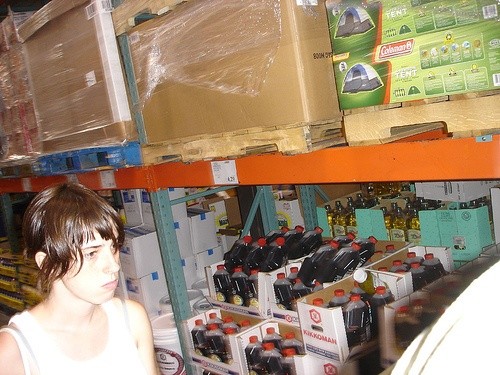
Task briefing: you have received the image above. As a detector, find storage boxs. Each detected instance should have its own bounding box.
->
[0,0,500,375]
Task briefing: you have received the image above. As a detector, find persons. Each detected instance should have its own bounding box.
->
[0,182,163,375]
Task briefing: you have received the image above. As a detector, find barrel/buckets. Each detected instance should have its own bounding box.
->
[151,312,187,375]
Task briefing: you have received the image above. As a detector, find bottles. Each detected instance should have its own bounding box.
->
[325,184,494,247]
[192,223,447,375]
